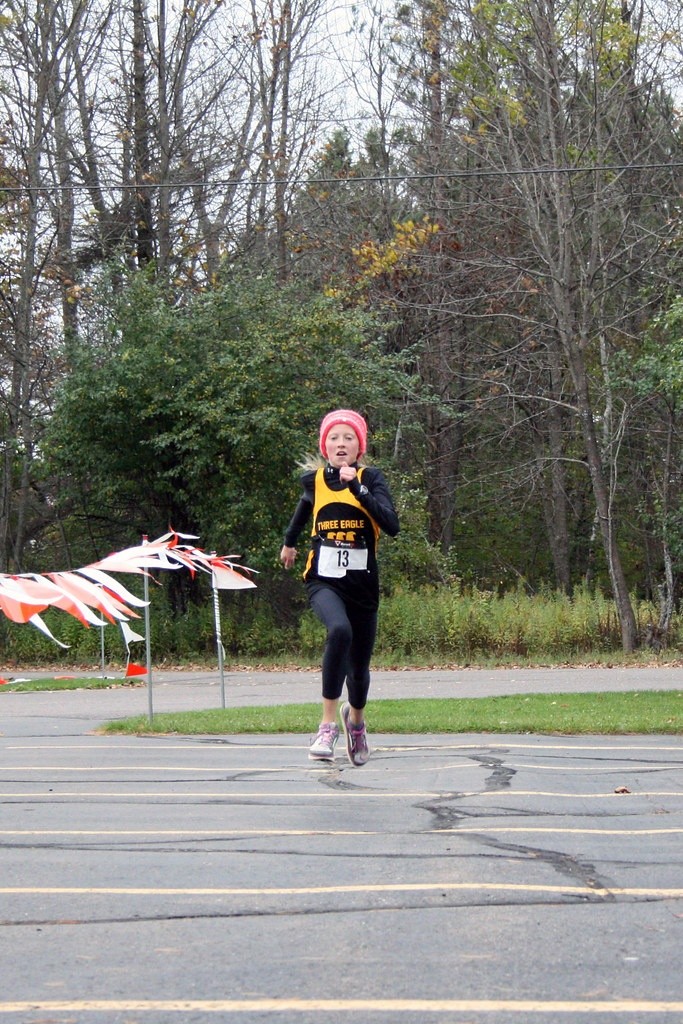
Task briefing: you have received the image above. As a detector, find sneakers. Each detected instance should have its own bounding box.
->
[307,721,339,762]
[340,700,371,768]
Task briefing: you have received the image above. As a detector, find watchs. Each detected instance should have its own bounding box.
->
[356,485,369,500]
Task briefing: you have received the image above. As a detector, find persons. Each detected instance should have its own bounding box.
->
[280,409,400,766]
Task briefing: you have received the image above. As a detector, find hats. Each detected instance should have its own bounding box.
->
[319,409,368,461]
